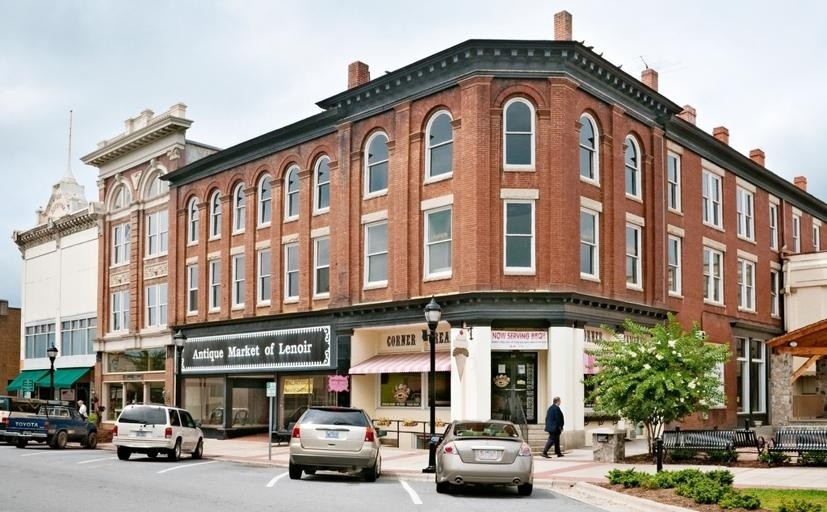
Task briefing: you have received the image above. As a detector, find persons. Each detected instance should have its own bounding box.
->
[77,400,88,422]
[541,396,565,459]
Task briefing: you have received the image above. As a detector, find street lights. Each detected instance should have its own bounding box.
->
[421,293,442,474]
[169,328,188,409]
[45,342,58,402]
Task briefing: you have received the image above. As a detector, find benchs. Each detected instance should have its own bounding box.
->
[652,430,736,466]
[736,430,766,456]
[767,430,827,468]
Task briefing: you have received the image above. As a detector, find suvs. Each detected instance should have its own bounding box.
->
[287,404,383,483]
[112,404,205,462]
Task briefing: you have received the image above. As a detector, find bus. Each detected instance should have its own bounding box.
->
[0,395,73,446]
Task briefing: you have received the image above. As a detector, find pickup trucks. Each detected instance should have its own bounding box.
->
[5,406,97,451]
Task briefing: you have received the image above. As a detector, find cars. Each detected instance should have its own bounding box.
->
[282,406,308,431]
[430,420,534,497]
